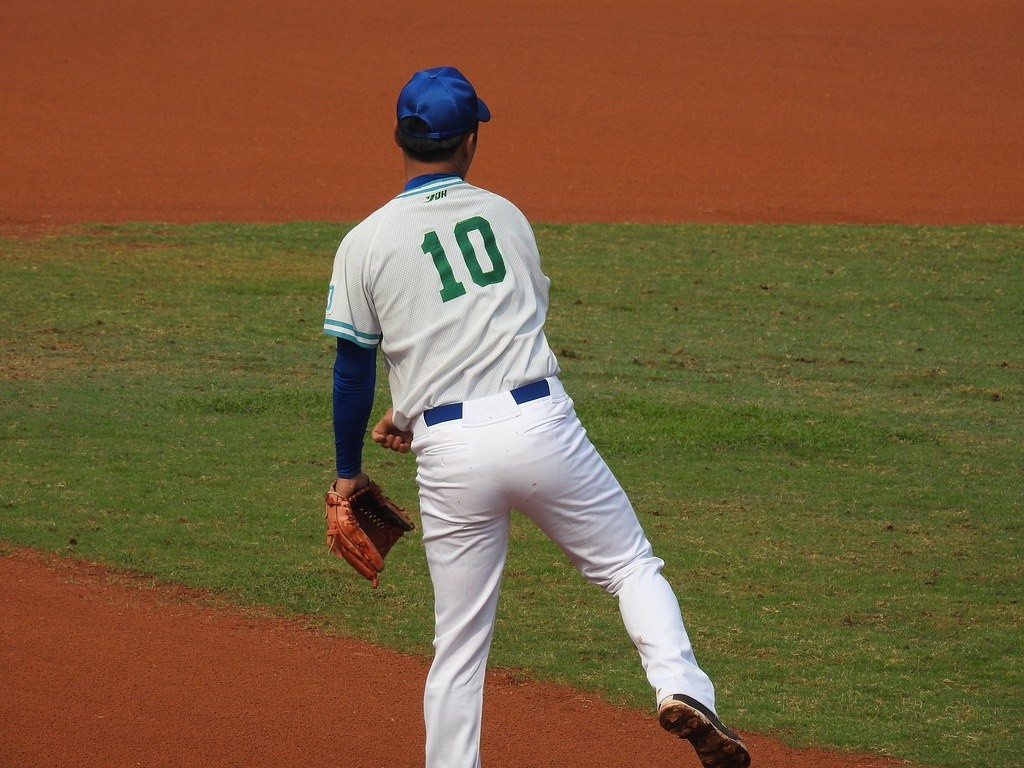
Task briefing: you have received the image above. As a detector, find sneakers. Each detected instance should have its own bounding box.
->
[657,694,751,768]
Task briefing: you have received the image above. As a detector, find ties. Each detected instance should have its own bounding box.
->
[397,65,491,138]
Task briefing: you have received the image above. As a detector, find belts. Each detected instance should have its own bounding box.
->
[424,377,549,427]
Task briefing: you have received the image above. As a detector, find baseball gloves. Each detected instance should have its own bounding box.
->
[322,476,417,589]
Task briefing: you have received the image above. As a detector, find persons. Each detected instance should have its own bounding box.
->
[324,67,751,768]
